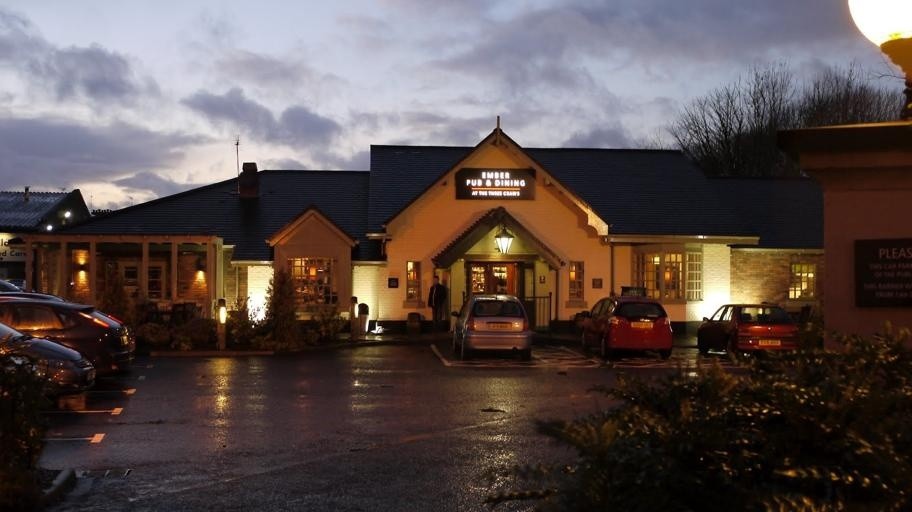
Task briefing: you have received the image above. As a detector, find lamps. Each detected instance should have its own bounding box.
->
[495,226,515,255]
[214,299,227,325]
[80,265,85,271]
[198,265,204,271]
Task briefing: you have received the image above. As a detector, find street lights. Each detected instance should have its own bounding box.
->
[837,1,912,130]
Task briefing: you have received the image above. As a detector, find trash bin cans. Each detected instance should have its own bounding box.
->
[407,312,420,335]
[359,303,369,334]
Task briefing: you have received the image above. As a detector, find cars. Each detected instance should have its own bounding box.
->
[1,279,138,493]
[452,291,531,359]
[575,294,676,361]
[695,301,798,356]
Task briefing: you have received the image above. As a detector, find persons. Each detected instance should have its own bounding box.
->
[428,275,449,332]
